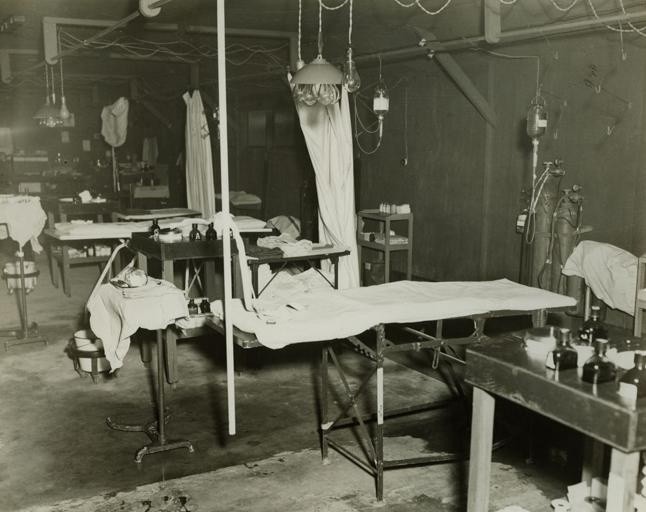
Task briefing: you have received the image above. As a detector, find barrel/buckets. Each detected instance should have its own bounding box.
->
[5,262,37,290]
[69,329,112,374]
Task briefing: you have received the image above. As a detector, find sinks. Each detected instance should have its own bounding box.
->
[610,340,646,374]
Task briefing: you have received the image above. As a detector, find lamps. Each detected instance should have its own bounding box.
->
[285,0,360,106]
[31,26,69,129]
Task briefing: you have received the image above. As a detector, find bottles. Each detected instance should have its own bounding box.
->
[582,337,616,384]
[189,223,202,242]
[618,350,645,411]
[206,224,217,240]
[150,219,160,235]
[372,87,390,120]
[545,328,578,371]
[187,298,199,315]
[577,304,609,346]
[526,104,547,145]
[200,299,211,313]
